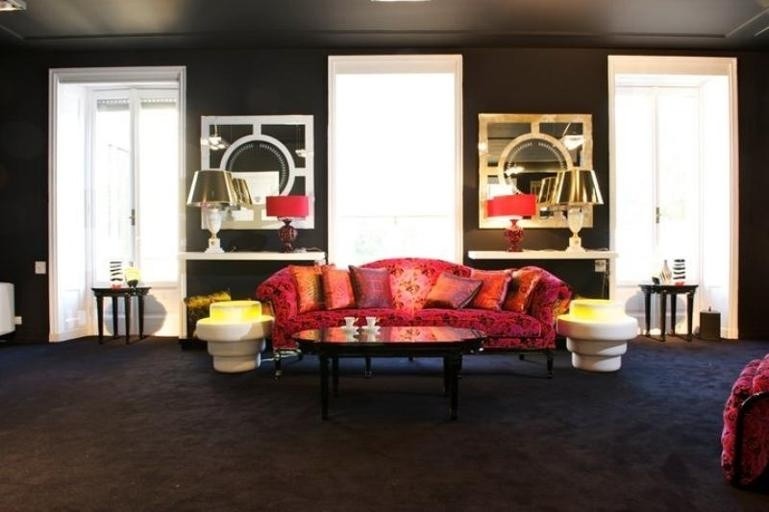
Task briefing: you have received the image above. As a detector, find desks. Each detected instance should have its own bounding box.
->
[90,285,151,345]
[637,281,700,342]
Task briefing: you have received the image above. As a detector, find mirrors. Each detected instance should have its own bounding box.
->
[200,112,316,233]
[476,111,597,232]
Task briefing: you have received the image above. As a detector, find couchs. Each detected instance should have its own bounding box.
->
[255,255,572,381]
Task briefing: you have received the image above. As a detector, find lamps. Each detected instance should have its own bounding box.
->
[185,167,240,253]
[264,194,310,252]
[484,193,539,251]
[226,178,254,224]
[196,298,275,377]
[555,298,640,375]
[538,175,566,222]
[544,167,604,253]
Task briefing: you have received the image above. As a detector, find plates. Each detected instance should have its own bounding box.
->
[361,324,381,332]
[341,325,358,332]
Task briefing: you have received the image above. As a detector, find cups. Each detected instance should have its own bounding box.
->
[366,316,380,327]
[343,315,358,327]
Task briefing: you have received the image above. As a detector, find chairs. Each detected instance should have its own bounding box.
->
[718,353,769,512]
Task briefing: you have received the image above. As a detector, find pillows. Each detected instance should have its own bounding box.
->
[288,262,324,317]
[470,268,514,313]
[424,271,484,310]
[348,264,398,310]
[319,264,358,311]
[502,268,544,314]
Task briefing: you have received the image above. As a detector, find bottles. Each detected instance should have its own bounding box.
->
[124,262,140,288]
